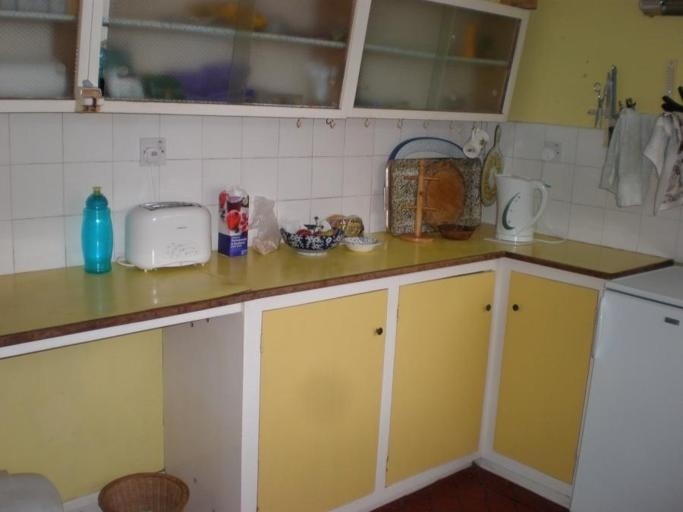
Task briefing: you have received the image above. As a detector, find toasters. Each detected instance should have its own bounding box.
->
[120,199,212,271]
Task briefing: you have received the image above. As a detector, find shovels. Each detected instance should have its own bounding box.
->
[481,126,505,205]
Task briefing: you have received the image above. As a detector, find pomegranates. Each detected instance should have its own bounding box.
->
[227,209,241,229]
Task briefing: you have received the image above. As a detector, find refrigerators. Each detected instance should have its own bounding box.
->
[567,265,683,512]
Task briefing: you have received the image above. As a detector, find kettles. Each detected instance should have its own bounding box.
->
[493,174,549,242]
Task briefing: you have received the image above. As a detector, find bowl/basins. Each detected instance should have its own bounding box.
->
[437,223,476,241]
[278,225,343,257]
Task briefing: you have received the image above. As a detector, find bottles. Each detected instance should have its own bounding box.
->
[78,184,115,274]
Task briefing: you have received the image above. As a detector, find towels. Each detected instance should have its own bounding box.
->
[600,109,683,214]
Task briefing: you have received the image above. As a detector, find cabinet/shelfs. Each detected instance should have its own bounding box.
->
[1,4,91,107]
[491,263,600,507]
[383,263,496,499]
[90,3,359,114]
[347,3,527,115]
[248,286,389,507]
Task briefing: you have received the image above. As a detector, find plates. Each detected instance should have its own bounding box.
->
[342,237,382,252]
[416,160,466,231]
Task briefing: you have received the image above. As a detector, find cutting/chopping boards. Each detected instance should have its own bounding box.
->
[384,156,484,236]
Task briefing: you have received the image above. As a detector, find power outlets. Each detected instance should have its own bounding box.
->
[543,141,561,164]
[139,137,167,166]
